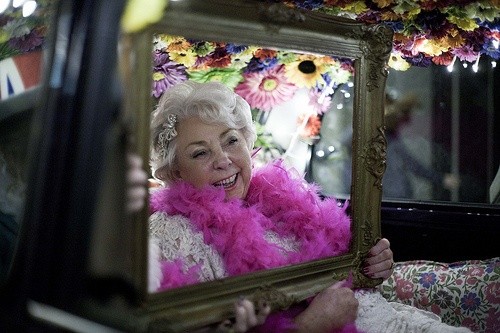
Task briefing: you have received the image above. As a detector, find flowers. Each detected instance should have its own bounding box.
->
[0,0,500,155]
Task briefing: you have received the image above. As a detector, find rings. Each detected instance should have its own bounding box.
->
[390,263,395,271]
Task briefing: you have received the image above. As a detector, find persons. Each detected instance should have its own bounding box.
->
[149,81,500,333]
[384,83,458,200]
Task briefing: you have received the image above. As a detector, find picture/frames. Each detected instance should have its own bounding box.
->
[116,0,395,333]
